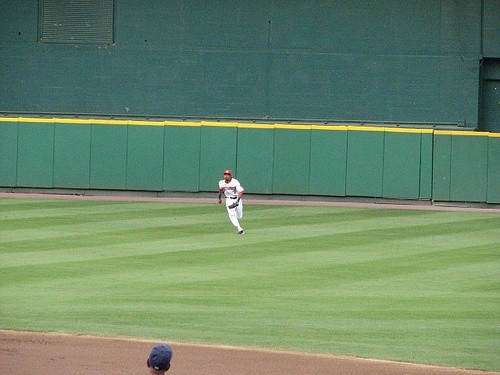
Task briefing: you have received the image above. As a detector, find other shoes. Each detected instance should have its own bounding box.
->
[239,228,243,233]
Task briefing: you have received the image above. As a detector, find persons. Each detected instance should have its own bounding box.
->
[218,170,246,235]
[147,344,173,375]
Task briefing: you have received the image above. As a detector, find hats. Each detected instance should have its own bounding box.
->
[149,344,172,368]
[224,170,232,174]
